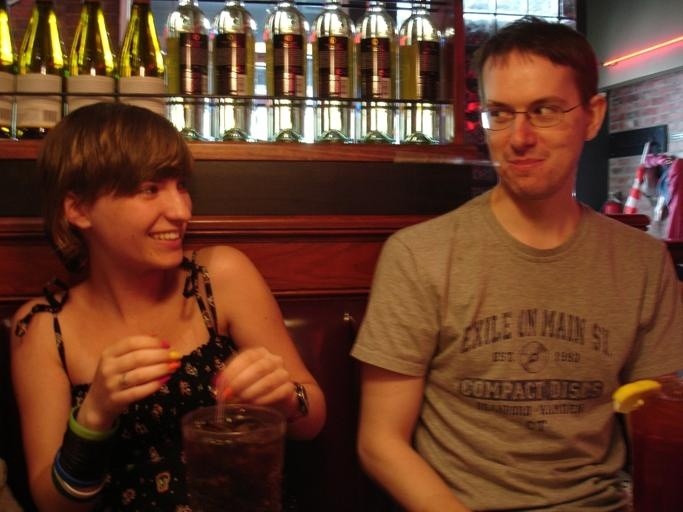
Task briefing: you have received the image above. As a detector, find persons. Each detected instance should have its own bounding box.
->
[357,16,683,507]
[8,102,328,506]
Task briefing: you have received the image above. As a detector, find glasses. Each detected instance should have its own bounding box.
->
[478,95,588,132]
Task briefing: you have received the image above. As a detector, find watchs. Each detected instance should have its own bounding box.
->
[293,381,312,416]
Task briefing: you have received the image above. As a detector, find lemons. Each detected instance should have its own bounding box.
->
[611,380,662,413]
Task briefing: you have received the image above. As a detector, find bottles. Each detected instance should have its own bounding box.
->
[312,0,356,145]
[0,0,19,139]
[360,0,396,146]
[70,1,115,117]
[264,0,307,144]
[118,0,165,121]
[399,0,442,145]
[210,0,257,144]
[162,0,210,143]
[16,0,65,141]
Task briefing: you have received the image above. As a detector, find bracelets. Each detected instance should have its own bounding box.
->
[67,407,117,444]
[53,454,112,500]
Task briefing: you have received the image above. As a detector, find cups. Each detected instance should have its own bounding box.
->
[631,376,683,512]
[181,404,287,512]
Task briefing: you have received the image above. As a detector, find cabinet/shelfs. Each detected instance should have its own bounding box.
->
[0,1,479,167]
